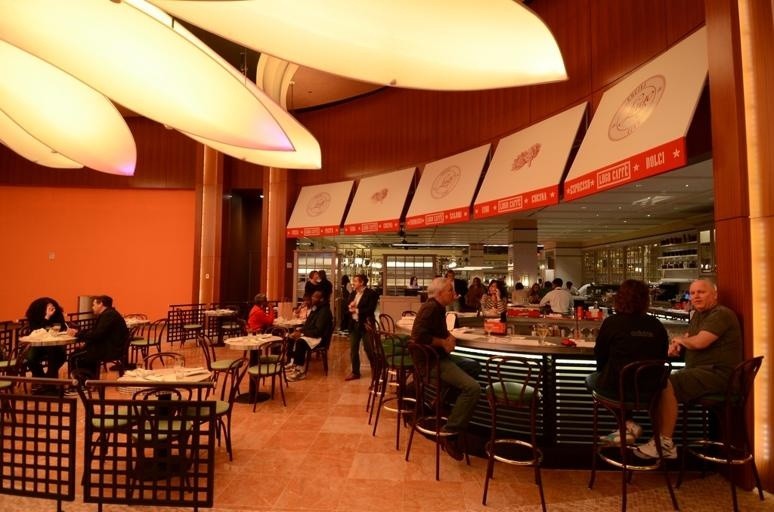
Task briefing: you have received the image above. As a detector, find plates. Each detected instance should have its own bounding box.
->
[229,337,243,342]
[128,369,151,376]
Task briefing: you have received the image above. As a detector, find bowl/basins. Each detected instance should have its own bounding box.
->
[264,333,272,338]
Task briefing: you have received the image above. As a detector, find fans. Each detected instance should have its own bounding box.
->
[384,221,420,252]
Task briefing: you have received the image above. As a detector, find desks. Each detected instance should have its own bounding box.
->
[19,334,79,396]
[117,367,214,482]
[396,307,563,356]
[201,310,237,348]
[223,334,284,404]
[125,318,151,339]
[272,316,307,363]
[258,325,290,387]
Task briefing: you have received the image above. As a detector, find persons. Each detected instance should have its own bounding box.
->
[249,294,288,336]
[282,271,333,382]
[632,275,745,461]
[28,296,68,391]
[344,275,380,380]
[62,296,128,396]
[585,280,672,444]
[341,275,353,329]
[408,272,578,322]
[411,277,480,461]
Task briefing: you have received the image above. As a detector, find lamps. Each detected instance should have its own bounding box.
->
[1,0,570,186]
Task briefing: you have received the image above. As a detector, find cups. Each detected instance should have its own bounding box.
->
[536,327,548,346]
[255,329,262,340]
[52,324,60,334]
[247,330,253,342]
[173,355,184,380]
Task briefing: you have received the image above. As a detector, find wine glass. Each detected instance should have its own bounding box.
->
[483,323,493,337]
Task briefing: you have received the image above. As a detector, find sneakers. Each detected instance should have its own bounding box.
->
[64,386,96,396]
[282,363,297,372]
[32,384,56,395]
[440,425,464,461]
[345,373,359,380]
[286,368,306,381]
[600,422,642,443]
[633,437,678,460]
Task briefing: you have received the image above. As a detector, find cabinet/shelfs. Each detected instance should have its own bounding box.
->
[653,231,701,284]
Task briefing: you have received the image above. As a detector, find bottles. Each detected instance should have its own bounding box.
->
[660,232,697,244]
[576,306,583,320]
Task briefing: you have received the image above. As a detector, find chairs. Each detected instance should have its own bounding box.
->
[400,345,471,481]
[222,306,242,339]
[363,315,407,414]
[123,313,148,358]
[141,352,188,411]
[130,317,170,367]
[579,356,686,512]
[71,369,142,486]
[482,351,556,512]
[80,326,139,390]
[3,347,28,422]
[247,338,286,412]
[178,357,251,464]
[672,350,769,512]
[198,333,240,402]
[363,325,420,448]
[378,313,413,391]
[128,386,197,503]
[1,342,32,394]
[304,317,337,377]
[176,308,204,347]
